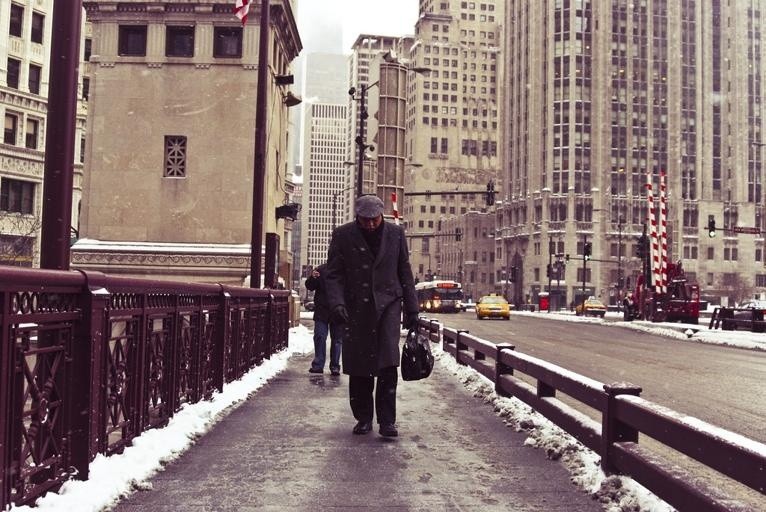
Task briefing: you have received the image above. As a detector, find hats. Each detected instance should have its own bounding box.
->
[354,195,385,218]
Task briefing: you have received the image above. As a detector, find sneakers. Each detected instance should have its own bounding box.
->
[353,421,373,435]
[378,422,398,437]
[331,370,340,376]
[308,368,323,374]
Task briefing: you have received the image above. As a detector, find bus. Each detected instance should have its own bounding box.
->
[414,279,463,313]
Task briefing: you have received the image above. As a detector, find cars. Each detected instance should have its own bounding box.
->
[576,295,607,318]
[734,301,766,330]
[304,301,315,311]
[475,293,510,320]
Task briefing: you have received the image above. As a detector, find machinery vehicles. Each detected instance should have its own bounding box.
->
[624,170,701,325]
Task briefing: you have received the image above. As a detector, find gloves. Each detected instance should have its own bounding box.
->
[329,305,350,327]
[405,313,418,333]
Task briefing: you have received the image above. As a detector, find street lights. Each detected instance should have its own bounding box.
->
[442,243,464,282]
[332,186,356,230]
[402,160,423,172]
[347,62,431,201]
[421,252,431,271]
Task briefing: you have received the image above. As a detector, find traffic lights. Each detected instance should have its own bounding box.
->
[585,241,592,256]
[708,215,715,238]
[509,267,515,280]
[621,278,624,288]
[566,254,569,263]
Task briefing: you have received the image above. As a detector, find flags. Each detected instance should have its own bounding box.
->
[233,0,252,28]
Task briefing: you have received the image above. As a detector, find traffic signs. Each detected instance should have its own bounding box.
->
[733,226,760,234]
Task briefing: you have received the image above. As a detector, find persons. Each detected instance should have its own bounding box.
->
[324,195,420,436]
[305,264,343,376]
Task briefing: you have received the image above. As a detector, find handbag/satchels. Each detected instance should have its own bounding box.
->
[401,334,435,382]
